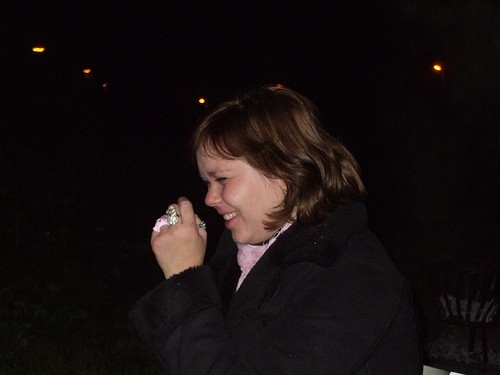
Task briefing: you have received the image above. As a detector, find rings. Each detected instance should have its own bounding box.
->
[166,206,179,226]
[197,221,206,230]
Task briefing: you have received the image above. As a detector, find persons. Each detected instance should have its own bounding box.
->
[125,86,424,375]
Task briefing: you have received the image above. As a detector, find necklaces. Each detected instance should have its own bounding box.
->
[251,227,281,246]
